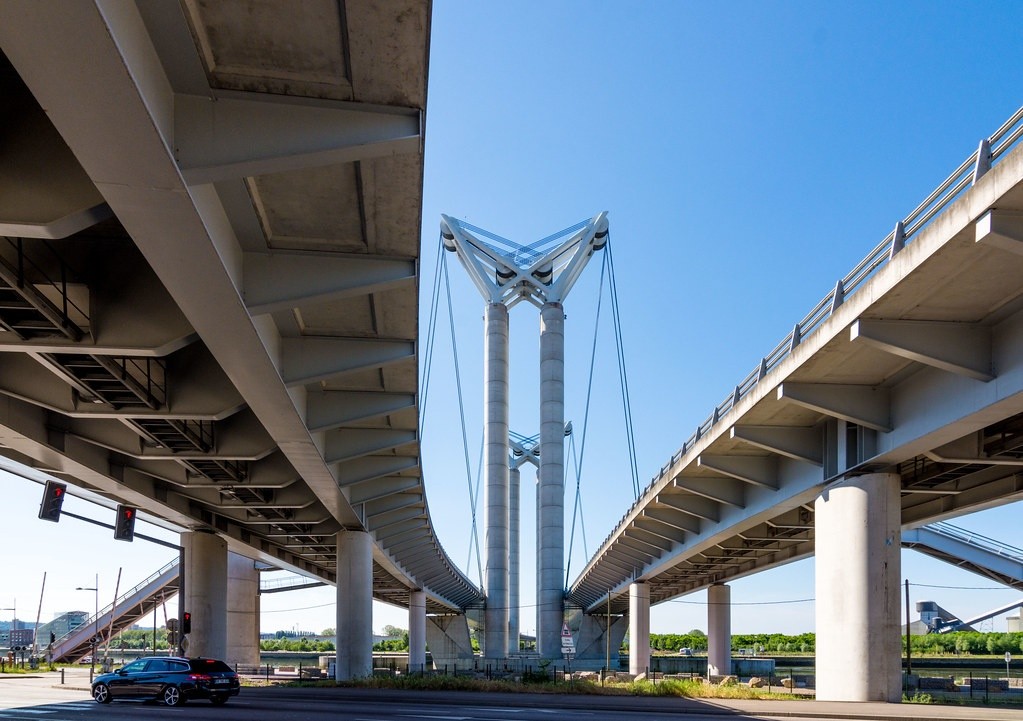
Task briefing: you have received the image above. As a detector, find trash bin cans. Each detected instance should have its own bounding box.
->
[102,658,112,673]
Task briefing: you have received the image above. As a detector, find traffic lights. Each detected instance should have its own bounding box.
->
[38,479,68,523]
[182,611,192,634]
[113,504,137,542]
[50,633,56,643]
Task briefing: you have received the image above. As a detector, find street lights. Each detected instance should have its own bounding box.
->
[150,594,161,657]
[0,597,17,664]
[75,573,99,672]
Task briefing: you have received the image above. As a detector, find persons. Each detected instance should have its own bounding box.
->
[136,656,141,665]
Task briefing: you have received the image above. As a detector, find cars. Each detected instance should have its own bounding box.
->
[89,653,241,707]
